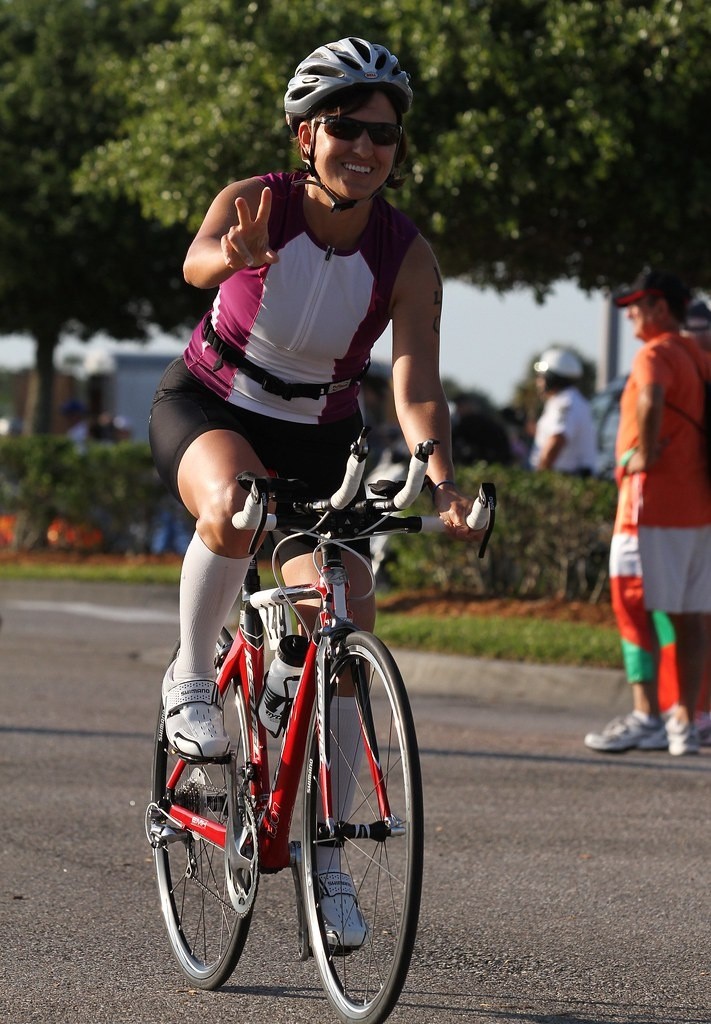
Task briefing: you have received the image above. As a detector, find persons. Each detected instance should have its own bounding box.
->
[60,398,136,450]
[148,37,485,957]
[356,265,711,757]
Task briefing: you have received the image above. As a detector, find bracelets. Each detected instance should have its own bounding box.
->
[428,480,455,506]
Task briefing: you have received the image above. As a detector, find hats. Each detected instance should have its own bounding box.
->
[680,303,711,332]
[612,271,693,305]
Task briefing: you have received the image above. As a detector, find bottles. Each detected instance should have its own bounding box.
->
[258,634,308,732]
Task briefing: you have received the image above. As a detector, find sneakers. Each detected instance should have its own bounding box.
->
[161,658,231,762]
[318,870,367,947]
[695,717,711,746]
[584,714,669,751]
[665,718,698,756]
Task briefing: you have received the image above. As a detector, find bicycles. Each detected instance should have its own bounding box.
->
[144,422,496,1024]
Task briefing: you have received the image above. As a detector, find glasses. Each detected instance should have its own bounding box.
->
[315,115,402,145]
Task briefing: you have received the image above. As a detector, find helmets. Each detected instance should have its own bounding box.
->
[534,348,583,379]
[284,37,413,137]
